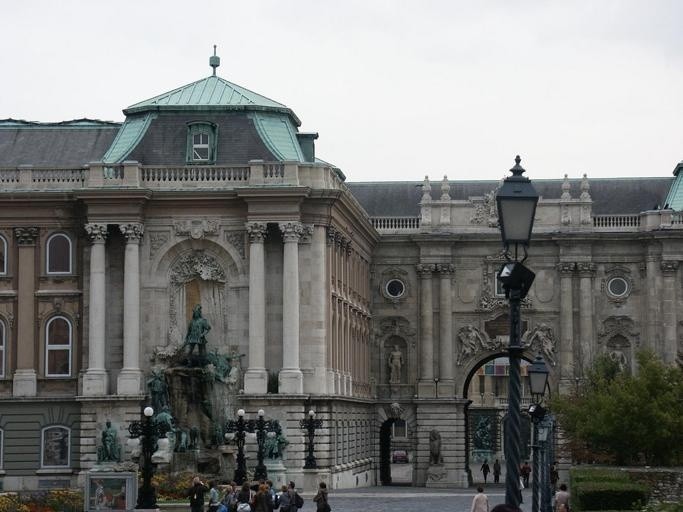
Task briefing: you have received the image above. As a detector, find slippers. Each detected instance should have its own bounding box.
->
[237,501,251,512]
[295,491,304,508]
[190,486,202,507]
[266,488,281,509]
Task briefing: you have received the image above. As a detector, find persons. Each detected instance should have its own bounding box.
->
[479,459,490,484]
[552,482,572,511]
[608,343,628,373]
[182,301,211,368]
[471,486,490,511]
[385,343,404,384]
[181,475,304,512]
[493,458,501,483]
[99,351,289,461]
[312,481,331,512]
[525,323,556,366]
[456,322,485,367]
[520,461,531,488]
[548,460,559,495]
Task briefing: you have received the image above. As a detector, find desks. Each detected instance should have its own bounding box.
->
[129,406,173,509]
[224,410,276,486]
[299,411,322,468]
[497,156,556,511]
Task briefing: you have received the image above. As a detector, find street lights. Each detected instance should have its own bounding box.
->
[325,503,332,512]
